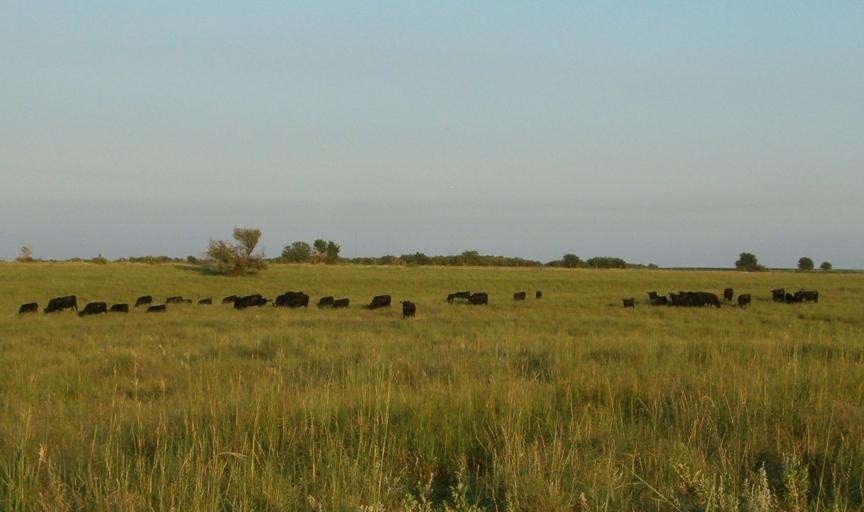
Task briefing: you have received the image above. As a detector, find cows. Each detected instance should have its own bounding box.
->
[514,292,526,300]
[769,288,819,304]
[43,291,309,317]
[367,295,392,311]
[400,299,416,319]
[536,291,542,298]
[623,298,634,310]
[724,288,734,304]
[646,291,722,308]
[446,291,470,304]
[316,296,334,310]
[331,298,349,308]
[737,293,751,308]
[469,292,488,306]
[18,302,39,314]
